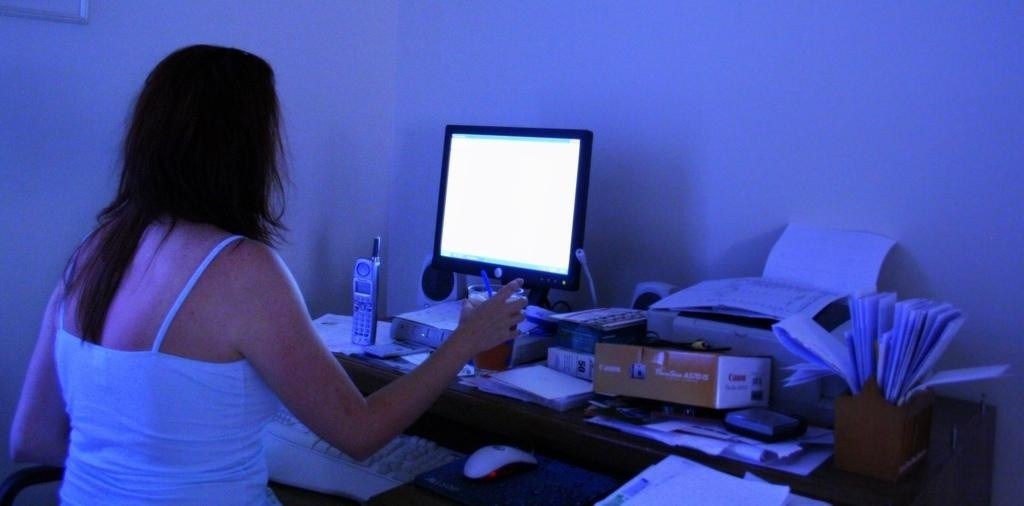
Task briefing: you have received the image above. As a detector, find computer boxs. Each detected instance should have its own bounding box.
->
[389,302,550,371]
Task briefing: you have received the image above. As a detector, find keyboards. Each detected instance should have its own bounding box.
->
[263,396,467,501]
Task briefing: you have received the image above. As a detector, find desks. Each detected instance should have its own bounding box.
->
[331,317,996,506]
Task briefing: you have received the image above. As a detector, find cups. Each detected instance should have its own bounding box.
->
[463,283,527,386]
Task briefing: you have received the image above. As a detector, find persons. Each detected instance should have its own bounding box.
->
[10,42,528,506]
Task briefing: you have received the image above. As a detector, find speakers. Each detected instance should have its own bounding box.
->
[419,253,463,309]
[631,281,673,313]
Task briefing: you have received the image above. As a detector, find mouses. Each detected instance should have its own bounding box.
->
[465,443,537,480]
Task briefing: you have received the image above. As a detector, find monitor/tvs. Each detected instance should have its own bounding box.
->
[433,124,593,311]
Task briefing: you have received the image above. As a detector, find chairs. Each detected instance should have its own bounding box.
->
[0,466,65,506]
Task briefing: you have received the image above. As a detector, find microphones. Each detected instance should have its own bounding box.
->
[576,248,601,303]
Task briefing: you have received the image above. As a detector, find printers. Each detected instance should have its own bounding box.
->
[646,277,857,423]
